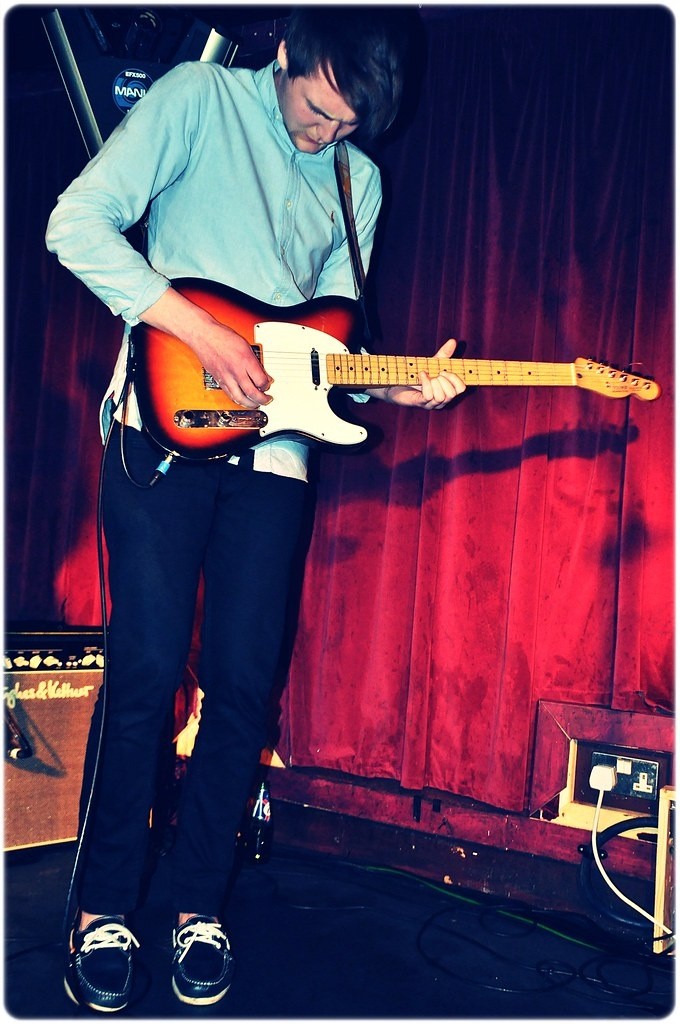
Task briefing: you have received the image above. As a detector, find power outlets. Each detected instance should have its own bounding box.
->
[591,753,658,800]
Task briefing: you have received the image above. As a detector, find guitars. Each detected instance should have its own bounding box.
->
[128,276,662,461]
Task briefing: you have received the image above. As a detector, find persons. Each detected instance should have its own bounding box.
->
[42,3,471,1012]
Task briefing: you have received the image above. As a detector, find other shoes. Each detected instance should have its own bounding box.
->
[172,913,234,1005]
[63,907,140,1012]
